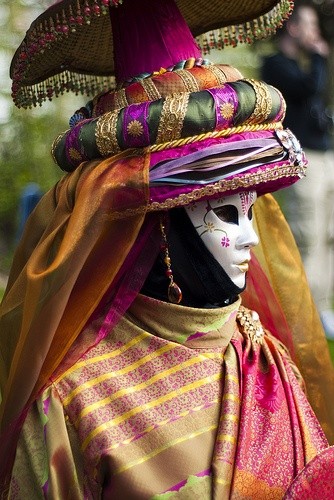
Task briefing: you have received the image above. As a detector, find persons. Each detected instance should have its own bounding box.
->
[260,2,334,342]
[0,0,334,500]
